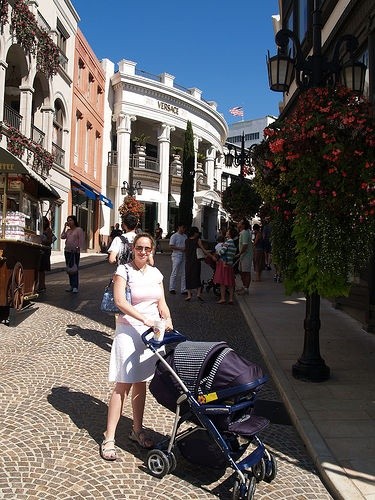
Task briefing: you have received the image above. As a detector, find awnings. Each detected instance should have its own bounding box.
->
[71,178,113,208]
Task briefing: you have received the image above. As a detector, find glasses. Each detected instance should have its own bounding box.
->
[133,246,152,252]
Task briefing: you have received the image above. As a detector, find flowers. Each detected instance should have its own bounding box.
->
[249,86,375,298]
[118,195,144,225]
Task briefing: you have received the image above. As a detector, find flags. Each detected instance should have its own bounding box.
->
[230,105,243,117]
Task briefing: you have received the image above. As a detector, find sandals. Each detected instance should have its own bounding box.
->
[101,438,117,460]
[128,429,153,448]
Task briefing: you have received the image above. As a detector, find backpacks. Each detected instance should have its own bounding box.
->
[118,235,133,265]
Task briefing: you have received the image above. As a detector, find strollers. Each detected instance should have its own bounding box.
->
[201,255,240,296]
[141,326,278,500]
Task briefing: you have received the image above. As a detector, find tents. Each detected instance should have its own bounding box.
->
[0,147,61,201]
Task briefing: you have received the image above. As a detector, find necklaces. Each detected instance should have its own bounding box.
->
[133,259,147,276]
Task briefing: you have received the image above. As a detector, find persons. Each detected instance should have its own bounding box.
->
[101,232,173,459]
[37,212,272,304]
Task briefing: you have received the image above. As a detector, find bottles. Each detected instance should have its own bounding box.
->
[0,210,30,241]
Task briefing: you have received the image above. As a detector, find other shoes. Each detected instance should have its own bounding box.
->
[73,288,79,293]
[65,285,72,292]
[185,297,192,301]
[274,277,282,283]
[236,287,249,295]
[197,295,204,301]
[216,300,226,304]
[181,291,192,296]
[169,290,176,294]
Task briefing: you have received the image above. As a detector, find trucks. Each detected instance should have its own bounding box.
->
[0,146,61,311]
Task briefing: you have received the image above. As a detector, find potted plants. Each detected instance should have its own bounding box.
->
[222,175,264,222]
[196,153,206,184]
[170,146,183,175]
[134,133,150,167]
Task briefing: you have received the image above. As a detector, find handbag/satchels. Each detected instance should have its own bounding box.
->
[196,248,207,260]
[66,254,78,274]
[100,264,131,316]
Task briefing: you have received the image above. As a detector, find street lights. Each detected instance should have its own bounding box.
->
[224,144,257,178]
[121,181,143,197]
[264,31,368,382]
